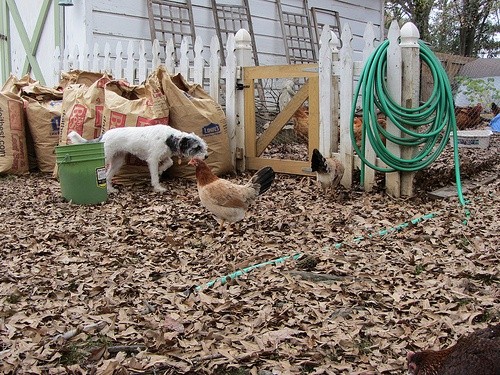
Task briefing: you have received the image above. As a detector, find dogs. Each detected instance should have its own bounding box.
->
[66,124,210,195]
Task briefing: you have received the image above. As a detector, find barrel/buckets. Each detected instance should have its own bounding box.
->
[53,141,108,204]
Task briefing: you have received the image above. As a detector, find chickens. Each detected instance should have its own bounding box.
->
[187,157,276,232]
[311,148,345,198]
[291,96,500,143]
